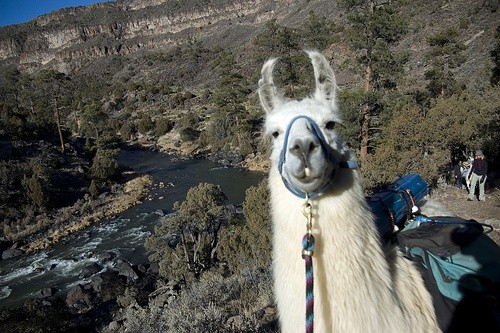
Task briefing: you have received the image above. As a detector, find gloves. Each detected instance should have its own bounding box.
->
[468,174,471,179]
[480,178,484,183]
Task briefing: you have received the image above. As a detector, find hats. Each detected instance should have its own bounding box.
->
[474,149,483,155]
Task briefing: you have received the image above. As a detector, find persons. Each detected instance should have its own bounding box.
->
[443,148,500,192]
[467,149,487,201]
